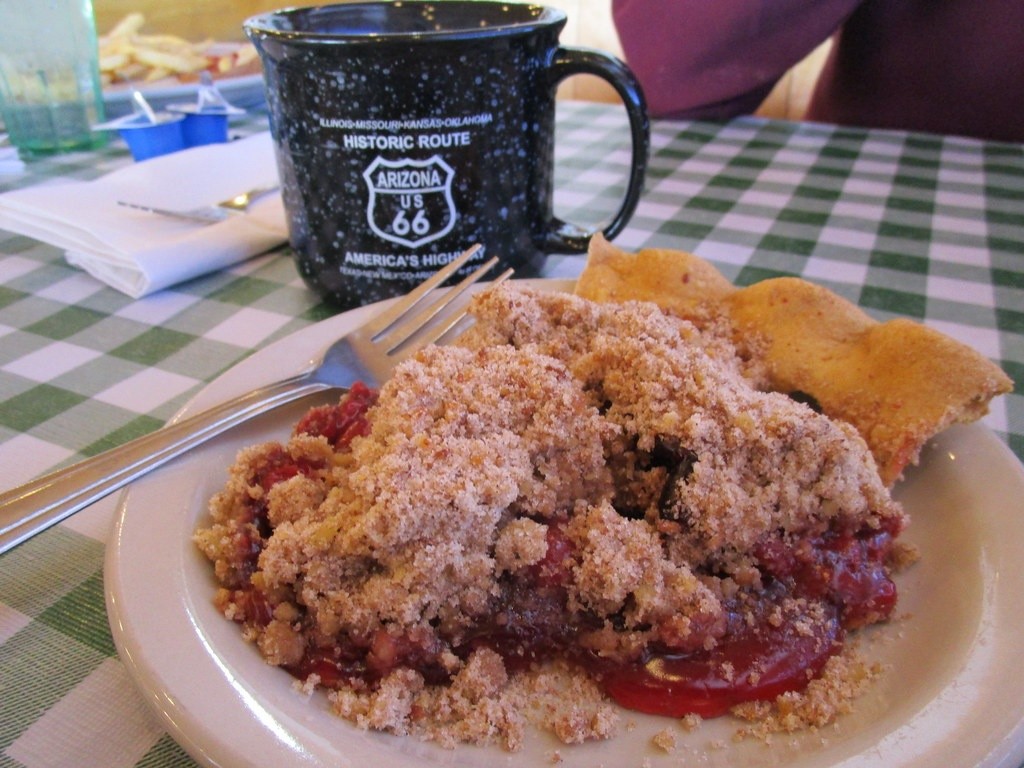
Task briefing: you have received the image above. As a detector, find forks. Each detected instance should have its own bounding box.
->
[0,243,516,560]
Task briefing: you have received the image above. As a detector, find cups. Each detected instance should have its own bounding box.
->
[1,2,108,165]
[92,101,247,162]
[242,0,649,312]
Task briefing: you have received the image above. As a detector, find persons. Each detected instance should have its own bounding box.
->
[548,0,1024,144]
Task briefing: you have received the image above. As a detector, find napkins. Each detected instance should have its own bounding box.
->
[0,122,290,298]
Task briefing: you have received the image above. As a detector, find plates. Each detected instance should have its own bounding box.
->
[105,42,270,120]
[103,276,1024,768]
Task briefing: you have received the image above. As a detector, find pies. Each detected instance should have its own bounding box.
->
[570,233,1014,490]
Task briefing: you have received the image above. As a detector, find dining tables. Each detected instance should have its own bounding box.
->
[0,85,1024,768]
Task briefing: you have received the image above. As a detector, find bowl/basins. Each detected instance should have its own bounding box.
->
[115,144,278,214]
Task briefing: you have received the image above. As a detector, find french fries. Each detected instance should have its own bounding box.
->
[8,11,257,104]
